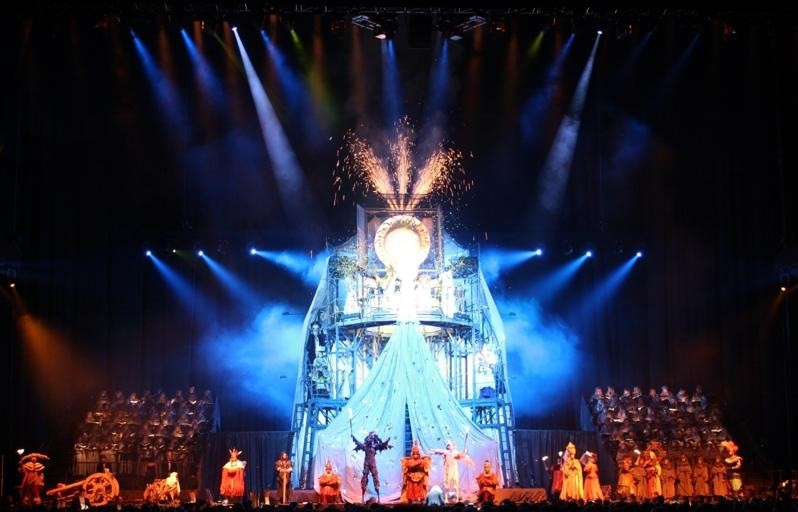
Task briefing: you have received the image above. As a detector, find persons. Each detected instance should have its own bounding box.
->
[306,322,328,397]
